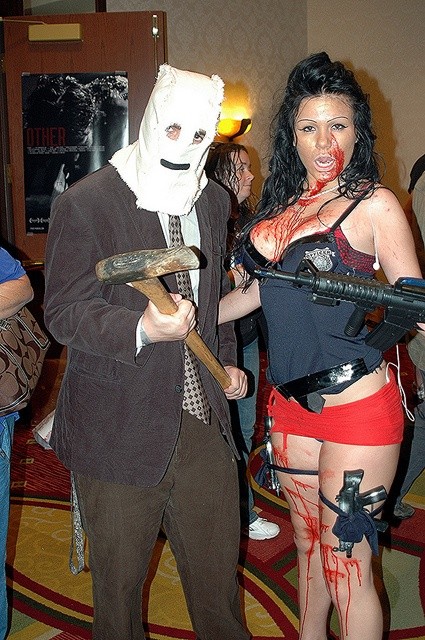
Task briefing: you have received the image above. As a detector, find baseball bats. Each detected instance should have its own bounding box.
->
[251,265,425,352]
[261,416,279,490]
[339,469,388,558]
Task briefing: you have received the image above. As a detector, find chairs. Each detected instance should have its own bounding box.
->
[241,516,280,540]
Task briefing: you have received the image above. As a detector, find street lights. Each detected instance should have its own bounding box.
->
[0,305,52,416]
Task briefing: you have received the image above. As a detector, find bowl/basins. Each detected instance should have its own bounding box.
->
[291,180,346,199]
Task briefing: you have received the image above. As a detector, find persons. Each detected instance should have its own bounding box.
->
[215,53,424,640]
[389,154,425,524]
[203,141,280,540]
[0,248,35,640]
[43,66,249,640]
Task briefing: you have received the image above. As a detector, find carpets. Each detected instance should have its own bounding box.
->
[167,214,211,425]
